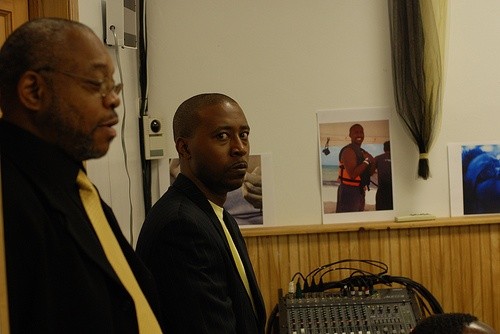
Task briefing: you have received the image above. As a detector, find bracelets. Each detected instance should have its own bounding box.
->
[363,160,369,166]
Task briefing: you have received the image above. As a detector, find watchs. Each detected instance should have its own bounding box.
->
[364,157,370,161]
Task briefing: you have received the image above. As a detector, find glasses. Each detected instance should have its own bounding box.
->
[36,68,124,99]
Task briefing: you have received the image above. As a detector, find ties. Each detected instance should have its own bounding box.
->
[76,170,163,334]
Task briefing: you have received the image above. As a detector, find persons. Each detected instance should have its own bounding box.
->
[136,93,266,334]
[0,18,160,334]
[462,148,500,209]
[336,124,393,213]
[407,313,495,334]
[169,158,263,226]
